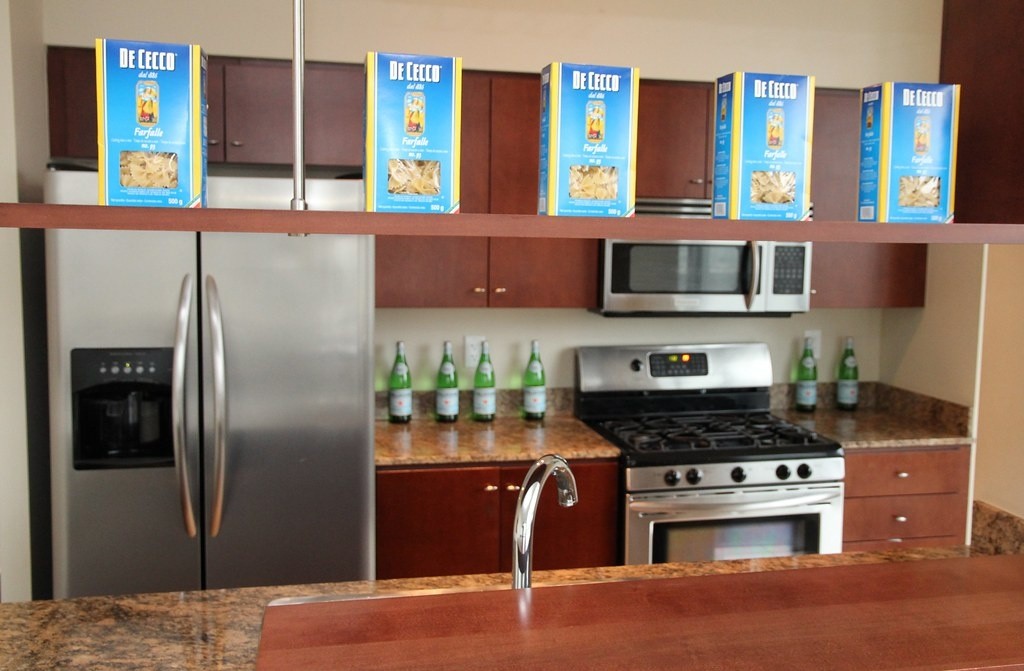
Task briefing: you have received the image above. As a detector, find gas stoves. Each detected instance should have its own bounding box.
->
[574,342,844,466]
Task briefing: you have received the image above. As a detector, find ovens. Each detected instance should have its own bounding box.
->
[624,481,846,565]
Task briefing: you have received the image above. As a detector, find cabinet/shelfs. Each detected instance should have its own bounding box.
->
[374,66,927,313]
[842,445,971,552]
[48,46,366,168]
[376,459,619,579]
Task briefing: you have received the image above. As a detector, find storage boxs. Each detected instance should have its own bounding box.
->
[361,51,463,214]
[856,81,961,224]
[94,38,208,209]
[711,70,815,222]
[537,62,640,220]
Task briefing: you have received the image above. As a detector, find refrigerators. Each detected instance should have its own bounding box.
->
[43,228,377,600]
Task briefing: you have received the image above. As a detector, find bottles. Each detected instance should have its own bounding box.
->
[472,340,496,422]
[522,340,546,420]
[836,336,859,411]
[434,340,460,423]
[388,342,412,423]
[796,338,817,411]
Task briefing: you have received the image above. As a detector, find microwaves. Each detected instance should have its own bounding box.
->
[596,238,813,315]
